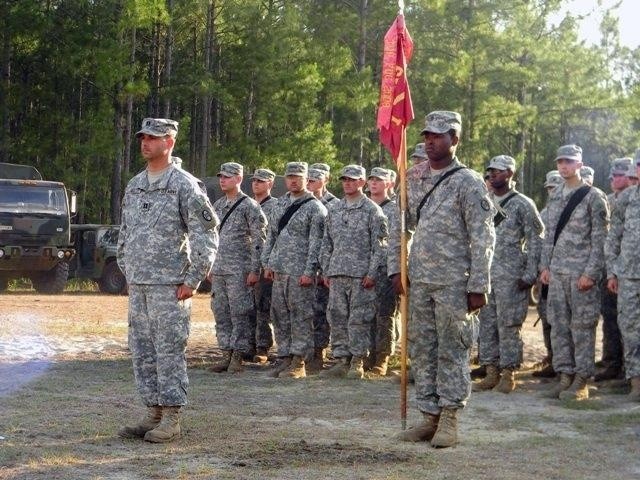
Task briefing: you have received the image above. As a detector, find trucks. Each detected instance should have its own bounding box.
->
[1,158,82,296]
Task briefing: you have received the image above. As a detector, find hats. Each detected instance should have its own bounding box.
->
[136,118,178,140]
[611,151,640,177]
[413,141,428,160]
[486,155,515,171]
[283,160,330,180]
[217,162,243,178]
[339,165,397,184]
[420,110,461,135]
[545,145,594,188]
[250,169,275,181]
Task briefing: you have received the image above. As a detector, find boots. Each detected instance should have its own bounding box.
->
[144,406,181,442]
[494,368,515,392]
[211,349,229,372]
[560,377,587,398]
[269,356,305,378]
[431,409,458,447]
[228,350,241,375]
[320,355,388,379]
[118,405,162,436]
[471,366,500,390]
[546,374,572,398]
[395,413,440,442]
[314,349,326,361]
[254,354,268,363]
[533,352,555,377]
[594,361,639,398]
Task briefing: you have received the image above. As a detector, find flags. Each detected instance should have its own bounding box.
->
[376,12,414,167]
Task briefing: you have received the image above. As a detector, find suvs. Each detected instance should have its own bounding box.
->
[67,219,129,298]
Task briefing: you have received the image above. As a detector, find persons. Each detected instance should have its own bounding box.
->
[208,161,397,380]
[470,144,640,402]
[116,118,221,444]
[410,143,428,165]
[388,111,496,447]
